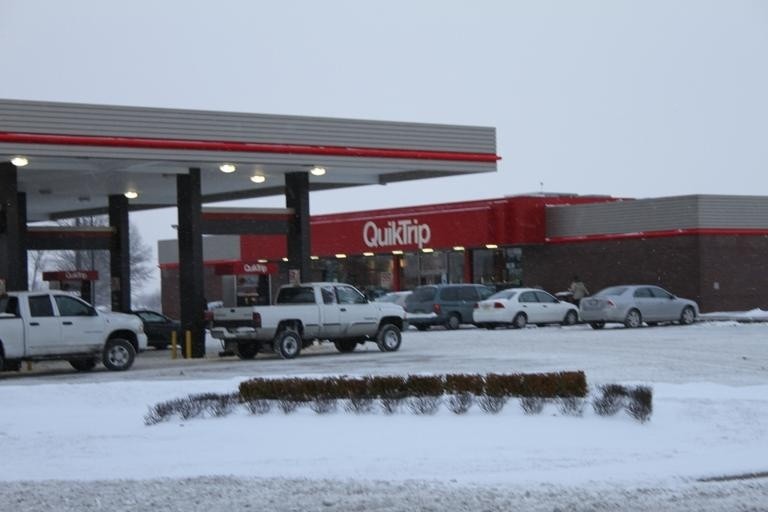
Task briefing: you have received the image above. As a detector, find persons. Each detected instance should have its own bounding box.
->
[567,274,589,307]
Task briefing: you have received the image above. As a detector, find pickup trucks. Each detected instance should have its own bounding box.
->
[214,281,406,361]
[0,290,149,372]
[203,296,215,331]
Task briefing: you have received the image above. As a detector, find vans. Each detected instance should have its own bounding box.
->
[406,282,496,330]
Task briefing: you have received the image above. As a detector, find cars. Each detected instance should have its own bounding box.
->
[587,284,700,327]
[371,289,412,310]
[474,287,579,329]
[134,308,181,350]
